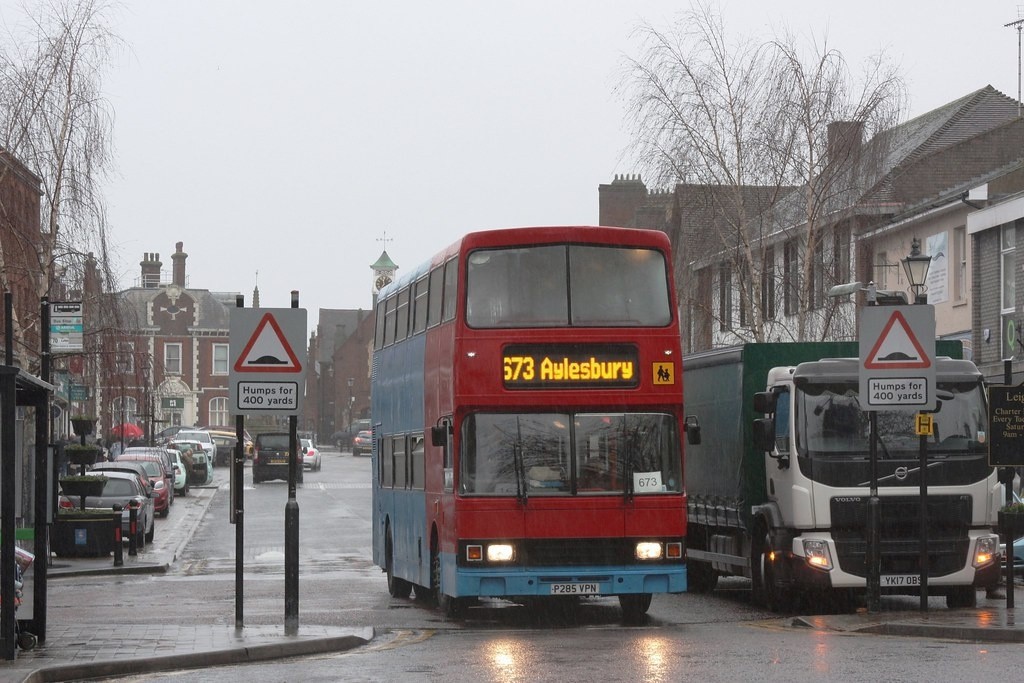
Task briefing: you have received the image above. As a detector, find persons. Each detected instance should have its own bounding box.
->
[982,553,1005,599]
[575,456,622,490]
[109,436,194,473]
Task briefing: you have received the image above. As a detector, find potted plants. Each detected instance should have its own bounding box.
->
[57,475,107,497]
[70,416,97,435]
[54,509,115,555]
[63,444,99,465]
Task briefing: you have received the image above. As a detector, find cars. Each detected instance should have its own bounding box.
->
[353,429,372,456]
[1000,535,1023,572]
[300,438,322,472]
[61,426,256,548]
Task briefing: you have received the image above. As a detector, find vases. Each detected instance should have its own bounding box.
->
[998,511,1024,534]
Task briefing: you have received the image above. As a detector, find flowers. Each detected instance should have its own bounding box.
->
[1001,502,1024,513]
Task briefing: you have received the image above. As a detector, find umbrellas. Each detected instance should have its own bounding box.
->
[111,423,145,442]
[331,431,349,439]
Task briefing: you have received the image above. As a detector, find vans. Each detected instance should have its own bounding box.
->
[249,430,309,484]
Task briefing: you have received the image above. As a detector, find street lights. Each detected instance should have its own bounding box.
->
[898,237,936,615]
[348,376,354,453]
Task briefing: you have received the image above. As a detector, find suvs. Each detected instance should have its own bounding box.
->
[330,417,371,446]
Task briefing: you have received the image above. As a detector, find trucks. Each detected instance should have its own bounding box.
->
[670,340,1004,616]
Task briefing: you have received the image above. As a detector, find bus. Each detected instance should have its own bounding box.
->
[367,226,702,623]
